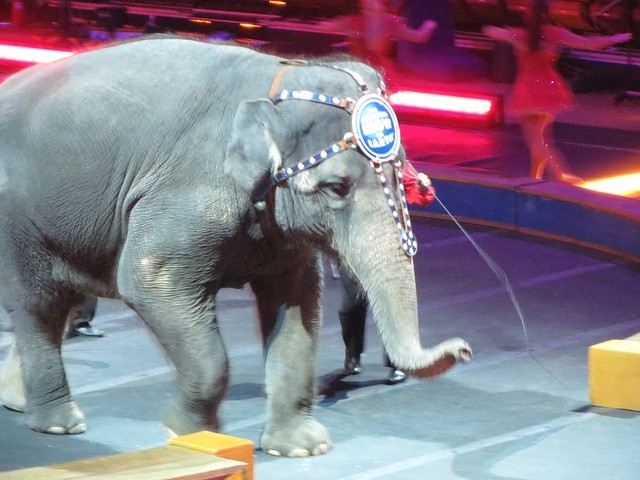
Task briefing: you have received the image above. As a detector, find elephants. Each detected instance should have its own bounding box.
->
[0,33,474,458]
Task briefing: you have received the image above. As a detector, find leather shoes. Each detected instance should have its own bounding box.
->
[69,322,104,337]
[345,346,362,374]
[383,367,407,385]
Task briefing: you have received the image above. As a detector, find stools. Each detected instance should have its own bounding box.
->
[0,444,249,479]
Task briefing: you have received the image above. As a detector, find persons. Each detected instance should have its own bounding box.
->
[336,264,408,384]
[66,295,105,339]
[481,0,632,187]
[318,3,436,99]
